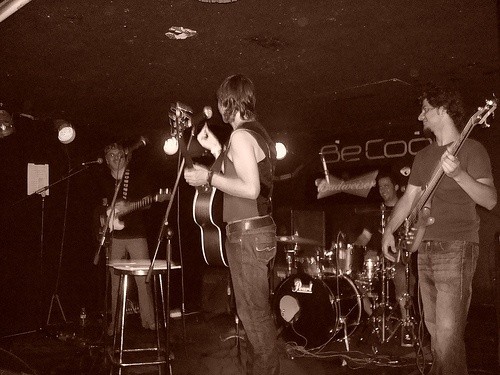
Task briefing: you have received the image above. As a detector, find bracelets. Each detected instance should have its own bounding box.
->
[207,171,214,187]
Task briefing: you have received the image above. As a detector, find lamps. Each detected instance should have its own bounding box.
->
[0,109,16,137]
[56,120,77,144]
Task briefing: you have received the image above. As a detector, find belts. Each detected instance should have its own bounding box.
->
[228,215,274,234]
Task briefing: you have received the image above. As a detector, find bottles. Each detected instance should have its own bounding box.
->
[79,308,87,328]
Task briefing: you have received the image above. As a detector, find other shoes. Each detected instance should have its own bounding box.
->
[144,320,161,329]
[102,327,121,335]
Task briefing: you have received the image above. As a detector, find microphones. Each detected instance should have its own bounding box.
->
[123,137,151,155]
[82,157,104,165]
[185,105,213,130]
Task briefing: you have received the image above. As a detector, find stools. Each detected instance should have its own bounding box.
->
[110,270,174,375]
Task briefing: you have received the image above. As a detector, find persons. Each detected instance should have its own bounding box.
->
[355,83,498,375]
[185,75,281,375]
[102,143,168,341]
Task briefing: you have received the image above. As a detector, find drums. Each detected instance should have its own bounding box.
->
[357,259,382,282]
[270,271,364,351]
[317,248,340,274]
[330,242,366,276]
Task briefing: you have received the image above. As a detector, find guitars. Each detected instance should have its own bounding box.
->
[396,92,499,253]
[167,100,230,268]
[99,186,173,237]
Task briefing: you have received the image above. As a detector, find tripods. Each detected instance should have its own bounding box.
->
[367,202,419,348]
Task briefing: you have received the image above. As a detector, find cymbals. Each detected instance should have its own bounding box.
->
[275,235,322,247]
